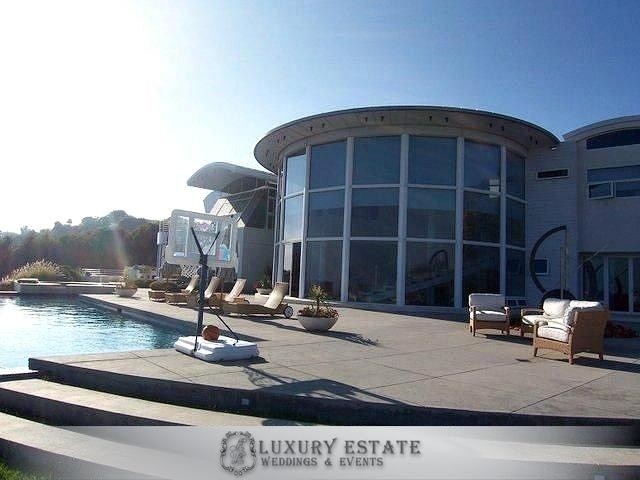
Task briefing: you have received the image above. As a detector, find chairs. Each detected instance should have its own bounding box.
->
[468,292,612,365]
[148,275,293,319]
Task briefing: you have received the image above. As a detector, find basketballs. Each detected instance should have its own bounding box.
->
[202,325,219,341]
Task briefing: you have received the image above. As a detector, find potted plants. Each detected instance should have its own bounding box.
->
[113,276,139,298]
[298,284,338,333]
